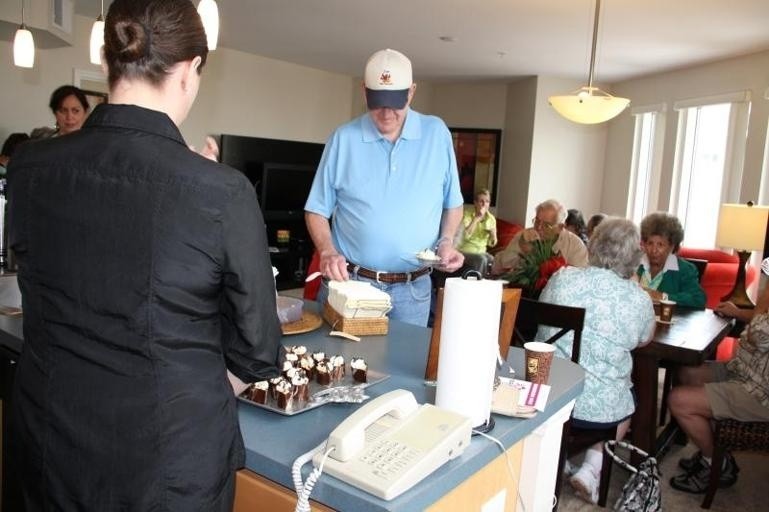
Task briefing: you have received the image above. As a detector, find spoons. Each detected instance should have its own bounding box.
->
[304,262,350,282]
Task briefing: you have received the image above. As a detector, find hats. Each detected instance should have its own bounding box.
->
[364,49,413,110]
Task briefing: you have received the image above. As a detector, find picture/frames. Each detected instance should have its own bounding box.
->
[448,127,502,206]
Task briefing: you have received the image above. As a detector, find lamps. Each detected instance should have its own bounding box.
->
[717,201,768,309]
[549,0,629,123]
[13,1,36,69]
[87,1,106,63]
[195,0,220,54]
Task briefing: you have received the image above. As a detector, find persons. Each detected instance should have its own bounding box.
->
[492,199,587,347]
[565,209,588,246]
[535,217,656,505]
[667,279,769,493]
[2,1,286,512]
[1,85,105,199]
[303,48,463,325]
[456,187,497,276]
[587,214,607,239]
[629,212,706,310]
[286,147,332,285]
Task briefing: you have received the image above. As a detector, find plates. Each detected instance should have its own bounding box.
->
[401,254,441,267]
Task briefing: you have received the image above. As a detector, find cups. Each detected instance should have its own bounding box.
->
[659,300,676,322]
[524,341,556,384]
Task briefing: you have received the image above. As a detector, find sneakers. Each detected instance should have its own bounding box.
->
[670,449,738,494]
[568,462,601,506]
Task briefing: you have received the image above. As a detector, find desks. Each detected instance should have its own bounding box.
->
[496,276,737,468]
[2,274,584,512]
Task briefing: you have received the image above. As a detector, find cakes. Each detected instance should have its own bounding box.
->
[248,343,368,409]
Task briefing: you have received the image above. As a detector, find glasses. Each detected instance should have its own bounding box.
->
[532,216,560,232]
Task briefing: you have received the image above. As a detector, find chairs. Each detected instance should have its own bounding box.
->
[517,297,617,511]
[700,418,769,509]
[681,247,756,313]
[495,216,522,253]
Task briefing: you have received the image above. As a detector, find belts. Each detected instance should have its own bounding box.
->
[346,261,430,284]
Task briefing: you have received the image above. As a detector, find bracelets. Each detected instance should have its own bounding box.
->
[436,234,453,249]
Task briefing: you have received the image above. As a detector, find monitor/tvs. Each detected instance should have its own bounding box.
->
[220,134,329,248]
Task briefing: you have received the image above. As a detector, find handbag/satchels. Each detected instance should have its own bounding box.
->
[605,440,662,511]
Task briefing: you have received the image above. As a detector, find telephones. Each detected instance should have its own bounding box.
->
[312,388,472,502]
